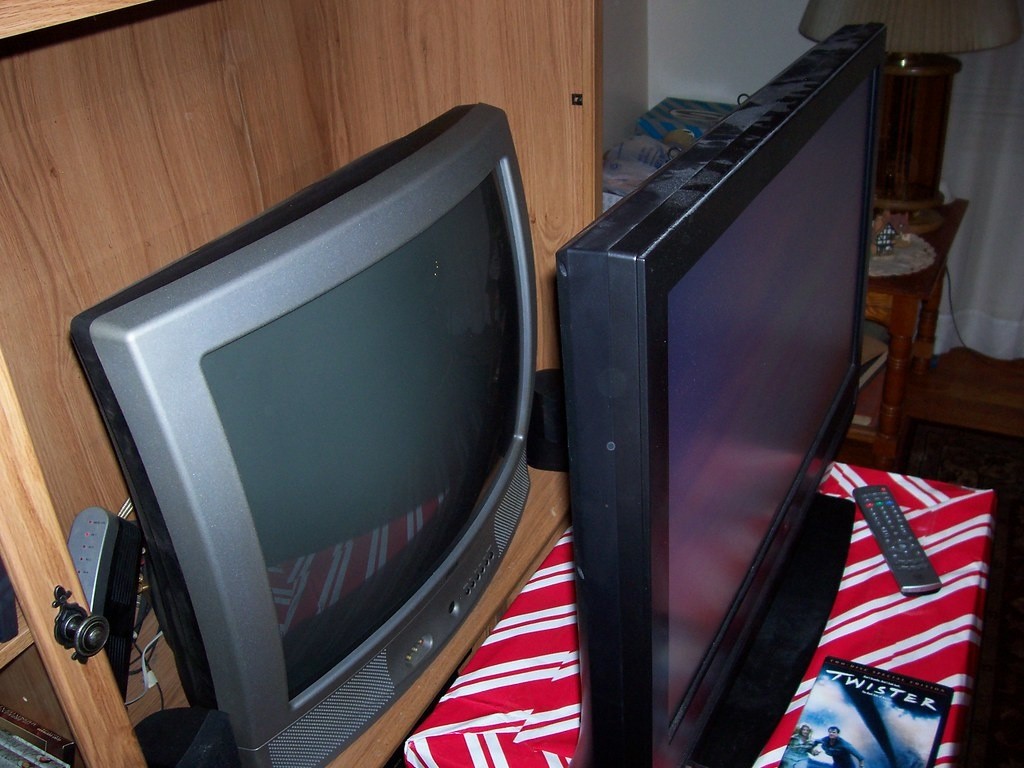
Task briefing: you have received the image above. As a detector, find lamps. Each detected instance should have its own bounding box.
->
[799,0,1024,234]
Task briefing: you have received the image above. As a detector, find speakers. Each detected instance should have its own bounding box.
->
[134,707,242,768]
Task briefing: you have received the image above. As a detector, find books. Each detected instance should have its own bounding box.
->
[858,336,888,389]
[0,707,74,768]
[0,559,18,643]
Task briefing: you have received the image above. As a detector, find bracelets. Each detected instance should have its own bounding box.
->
[859,765,864,768]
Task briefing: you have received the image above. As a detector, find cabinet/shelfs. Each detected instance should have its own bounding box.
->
[0,1,606,768]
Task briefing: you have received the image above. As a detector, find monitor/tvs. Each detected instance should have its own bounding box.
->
[72,100,539,768]
[557,20,890,768]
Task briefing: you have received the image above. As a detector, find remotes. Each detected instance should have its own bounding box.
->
[852,484,941,597]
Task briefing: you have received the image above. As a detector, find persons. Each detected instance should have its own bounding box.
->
[782,724,865,768]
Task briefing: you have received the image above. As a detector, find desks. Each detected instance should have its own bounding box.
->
[840,199,970,471]
[408,455,995,767]
